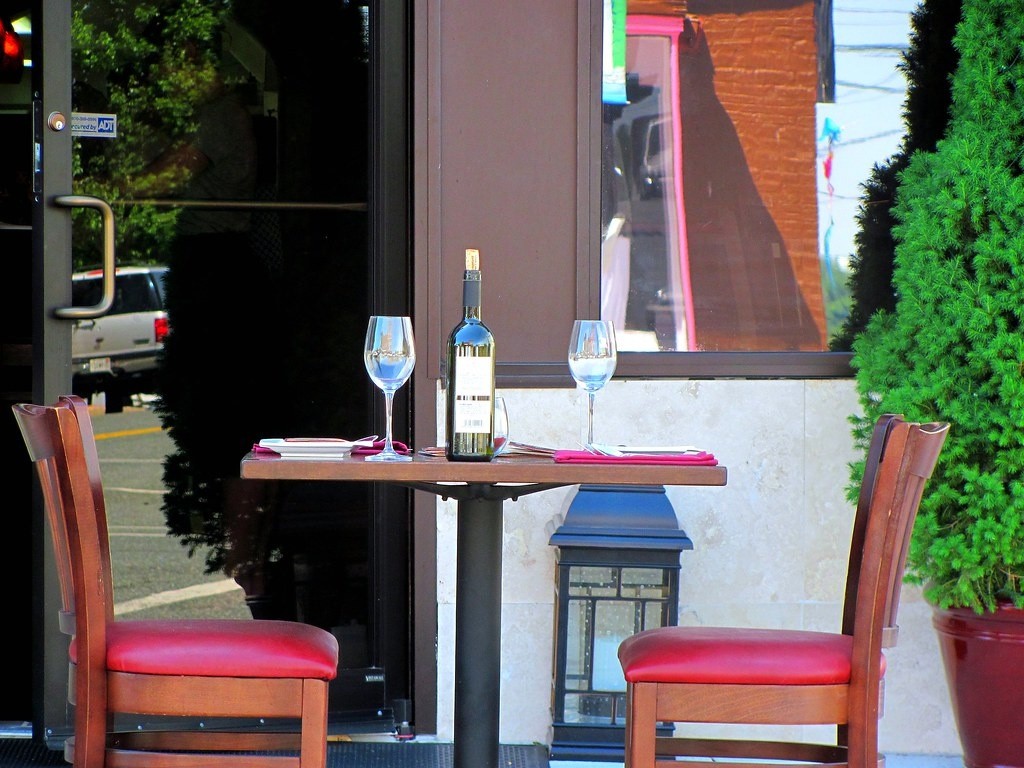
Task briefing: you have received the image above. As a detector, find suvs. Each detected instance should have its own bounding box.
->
[72,266,172,399]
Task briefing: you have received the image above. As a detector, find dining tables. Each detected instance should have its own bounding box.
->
[235,449,727,768]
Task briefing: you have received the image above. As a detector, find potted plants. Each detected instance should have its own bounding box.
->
[147,1,378,714]
[849,0,1024,766]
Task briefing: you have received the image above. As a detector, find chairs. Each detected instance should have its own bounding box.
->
[58,390,115,620]
[834,413,905,748]
[614,419,951,768]
[8,400,342,768]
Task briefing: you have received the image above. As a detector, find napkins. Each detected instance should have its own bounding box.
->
[554,448,719,467]
[250,440,409,456]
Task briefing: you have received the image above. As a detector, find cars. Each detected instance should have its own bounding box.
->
[640,116,670,201]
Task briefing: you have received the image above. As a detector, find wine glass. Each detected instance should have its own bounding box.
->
[364,316,416,461]
[568,320,617,452]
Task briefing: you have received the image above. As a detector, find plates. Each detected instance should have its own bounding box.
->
[606,446,702,455]
[260,442,356,459]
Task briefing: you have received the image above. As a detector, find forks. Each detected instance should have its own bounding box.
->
[575,441,604,456]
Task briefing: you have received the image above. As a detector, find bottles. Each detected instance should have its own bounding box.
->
[445,270,496,462]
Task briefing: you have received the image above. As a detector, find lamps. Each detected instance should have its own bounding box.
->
[546,481,693,768]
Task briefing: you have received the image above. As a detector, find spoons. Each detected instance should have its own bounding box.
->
[591,445,699,457]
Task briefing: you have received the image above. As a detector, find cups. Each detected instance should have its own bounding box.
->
[494,397,509,458]
